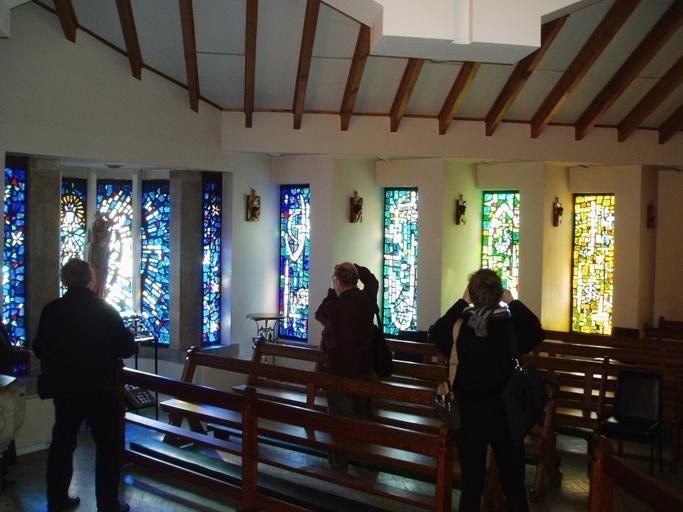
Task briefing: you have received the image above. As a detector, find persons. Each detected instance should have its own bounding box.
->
[32,258,135,511]
[0,315,34,368]
[315,263,394,473]
[430,269,546,512]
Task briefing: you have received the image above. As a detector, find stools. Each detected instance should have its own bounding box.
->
[247,314,282,365]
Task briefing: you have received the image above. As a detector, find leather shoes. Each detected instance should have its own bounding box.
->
[69,496,80,510]
[121,503,130,512]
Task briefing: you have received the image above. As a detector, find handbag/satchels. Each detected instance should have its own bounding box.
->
[38,330,56,400]
[503,316,549,439]
[432,319,464,427]
[366,290,395,364]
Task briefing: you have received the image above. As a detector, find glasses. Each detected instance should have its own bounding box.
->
[334,277,338,281]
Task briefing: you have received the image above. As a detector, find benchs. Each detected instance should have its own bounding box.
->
[120,367,452,512]
[232,335,561,492]
[383,338,683,478]
[161,346,498,512]
[519,329,683,459]
